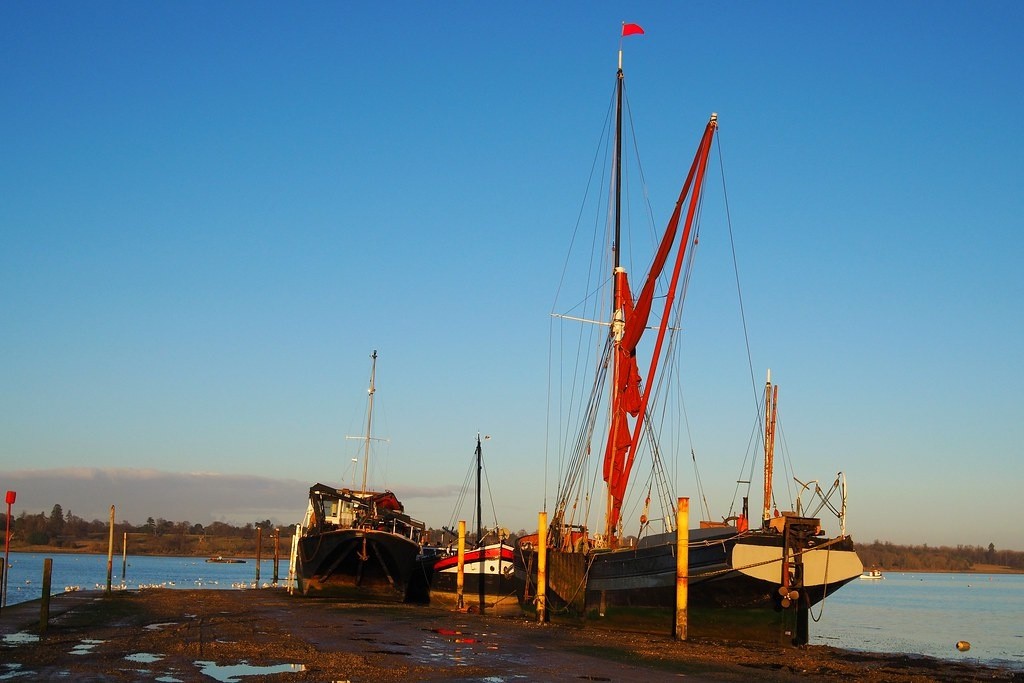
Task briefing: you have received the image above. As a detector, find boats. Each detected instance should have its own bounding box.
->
[204,556,248,564]
[860,570,885,580]
[286,350,438,605]
[428,430,526,607]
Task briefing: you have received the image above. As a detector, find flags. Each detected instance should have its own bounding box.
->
[622,24,644,36]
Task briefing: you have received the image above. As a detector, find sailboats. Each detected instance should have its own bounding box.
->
[528,18,859,622]
